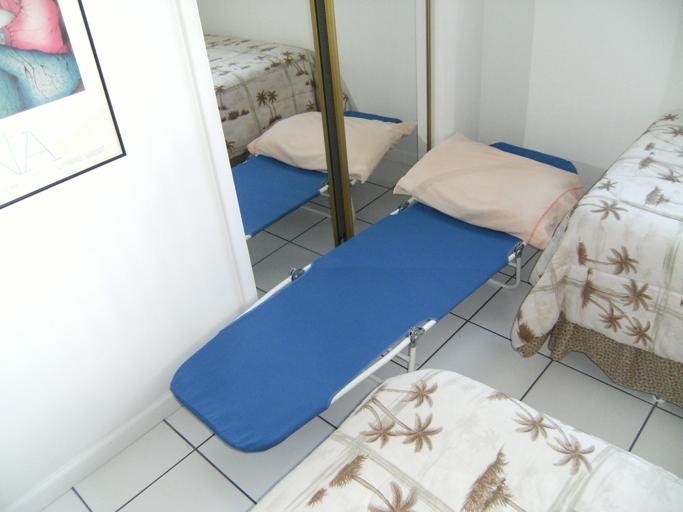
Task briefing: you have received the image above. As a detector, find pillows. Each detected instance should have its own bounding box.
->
[244,111,410,189]
[393,130,583,253]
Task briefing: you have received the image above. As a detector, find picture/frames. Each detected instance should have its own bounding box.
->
[0,0,128,210]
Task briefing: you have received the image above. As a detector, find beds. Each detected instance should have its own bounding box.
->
[232,371,683,512]
[552,109,683,379]
[201,30,315,164]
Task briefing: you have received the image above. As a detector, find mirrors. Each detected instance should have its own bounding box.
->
[333,1,428,239]
[195,0,334,308]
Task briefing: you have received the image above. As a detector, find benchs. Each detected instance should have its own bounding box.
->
[172,145,581,453]
[230,109,402,241]
